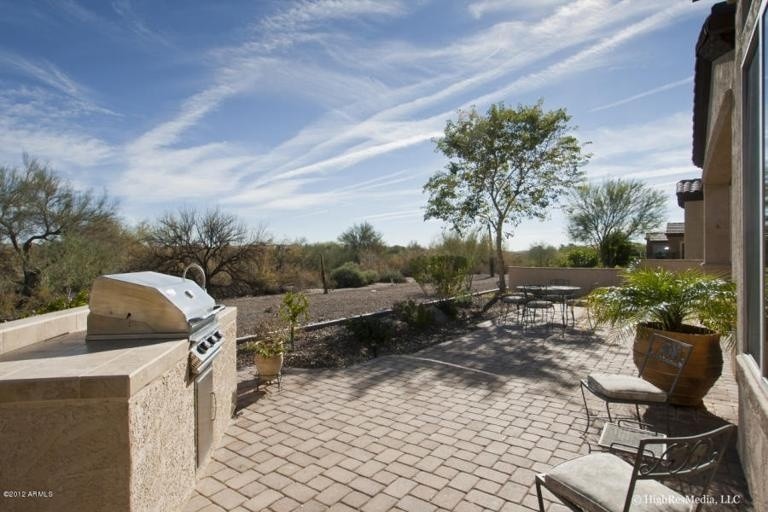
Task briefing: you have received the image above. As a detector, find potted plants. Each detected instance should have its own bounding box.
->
[579,259,739,408]
[248,338,285,376]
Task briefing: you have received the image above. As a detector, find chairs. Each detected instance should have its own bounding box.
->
[534,331,737,511]
[497,278,593,333]
